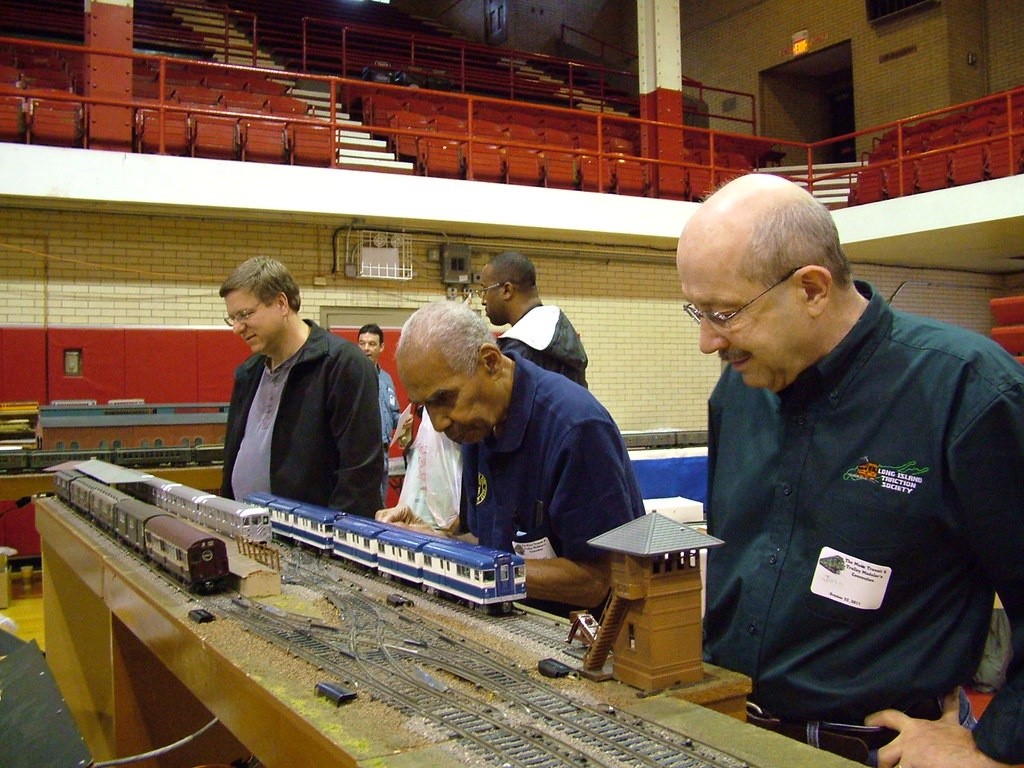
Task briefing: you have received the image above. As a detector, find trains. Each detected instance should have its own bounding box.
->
[0,443,225,476]
[243,491,528,617]
[127,466,272,548]
[54,469,230,595]
[620,427,709,450]
[0,397,145,409]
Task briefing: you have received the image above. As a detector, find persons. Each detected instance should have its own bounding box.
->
[216,255,386,526]
[474,247,594,393]
[674,167,1024,768]
[369,296,651,635]
[347,319,407,521]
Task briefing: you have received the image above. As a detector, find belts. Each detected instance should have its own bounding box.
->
[748,704,895,765]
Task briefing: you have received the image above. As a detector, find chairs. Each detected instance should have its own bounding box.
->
[0,0,1024,209]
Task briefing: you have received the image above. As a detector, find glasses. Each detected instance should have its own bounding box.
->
[475,282,502,303]
[223,300,259,326]
[683,268,801,326]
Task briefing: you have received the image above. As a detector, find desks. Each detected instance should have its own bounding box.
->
[33,493,865,768]
